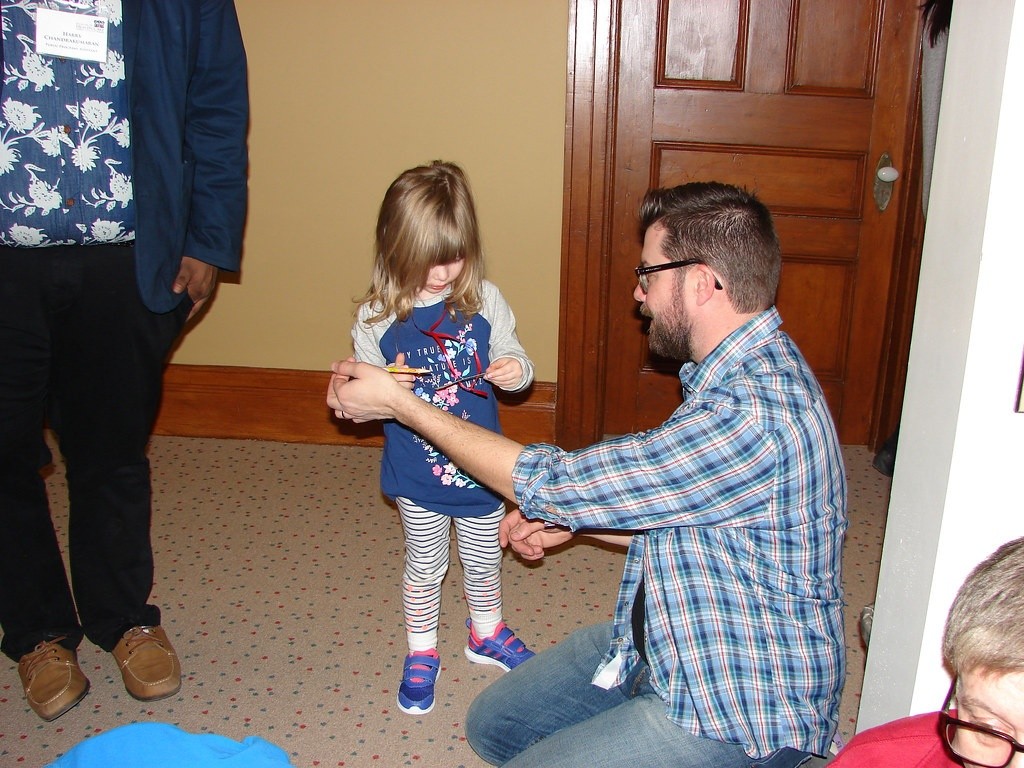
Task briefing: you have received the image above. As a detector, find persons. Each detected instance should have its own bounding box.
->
[322,179,851,768]
[0,0,252,725]
[349,158,538,717]
[822,535,1024,768]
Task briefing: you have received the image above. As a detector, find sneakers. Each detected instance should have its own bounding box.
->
[396,648,441,715]
[464,618,537,673]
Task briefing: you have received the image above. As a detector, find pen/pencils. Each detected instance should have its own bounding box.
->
[381,366,435,374]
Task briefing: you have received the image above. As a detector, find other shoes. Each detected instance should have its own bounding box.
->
[111,624,182,702]
[18,635,90,722]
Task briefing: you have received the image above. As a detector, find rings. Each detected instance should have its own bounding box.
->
[341,410,346,419]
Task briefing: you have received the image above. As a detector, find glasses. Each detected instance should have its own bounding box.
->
[939,674,1024,768]
[634,259,723,294]
